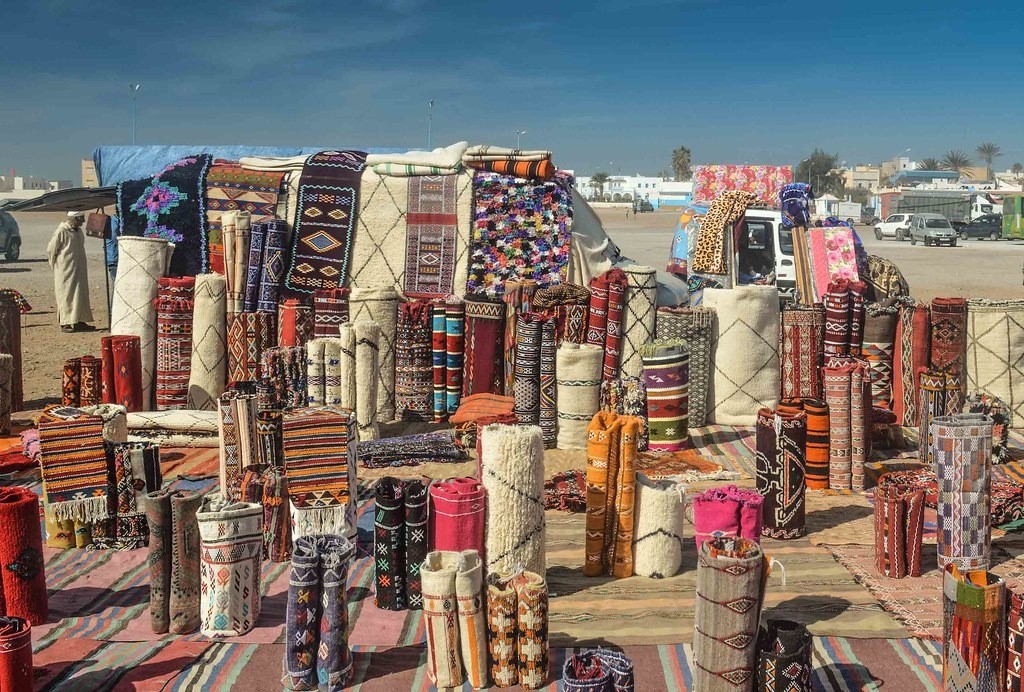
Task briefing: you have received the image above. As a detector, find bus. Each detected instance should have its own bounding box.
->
[1002,192,1024,242]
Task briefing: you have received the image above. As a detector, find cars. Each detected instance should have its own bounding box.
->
[633,199,654,214]
[0,211,23,262]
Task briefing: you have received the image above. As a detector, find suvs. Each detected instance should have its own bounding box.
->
[958,212,1003,241]
[874,213,914,241]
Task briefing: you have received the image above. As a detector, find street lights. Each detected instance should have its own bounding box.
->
[427,100,433,151]
[126,81,142,147]
[517,130,526,149]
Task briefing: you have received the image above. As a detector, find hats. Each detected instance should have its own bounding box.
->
[66,209,85,220]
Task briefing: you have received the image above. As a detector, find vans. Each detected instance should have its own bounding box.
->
[910,211,957,248]
[730,204,796,303]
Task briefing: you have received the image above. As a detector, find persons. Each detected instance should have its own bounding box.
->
[46,209,96,333]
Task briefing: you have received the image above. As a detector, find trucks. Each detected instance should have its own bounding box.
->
[896,194,994,233]
[831,201,878,227]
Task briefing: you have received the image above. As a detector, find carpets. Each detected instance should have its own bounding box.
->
[0,142,1024,692]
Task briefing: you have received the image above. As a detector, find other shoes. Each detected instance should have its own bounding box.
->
[59,323,95,334]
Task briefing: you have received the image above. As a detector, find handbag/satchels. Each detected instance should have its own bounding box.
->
[86,202,113,242]
